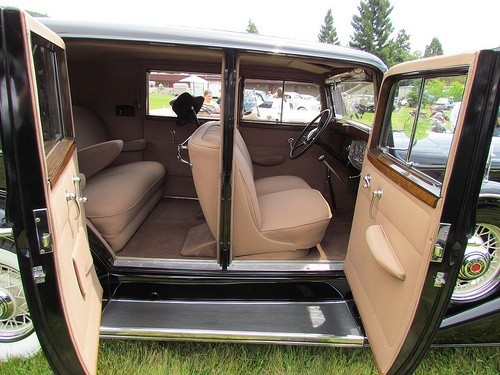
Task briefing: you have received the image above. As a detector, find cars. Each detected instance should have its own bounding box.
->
[350,92,456,114]
[0,5,500,375]
[215,88,321,113]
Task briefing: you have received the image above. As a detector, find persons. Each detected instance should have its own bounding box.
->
[270,87,289,125]
[200,90,221,115]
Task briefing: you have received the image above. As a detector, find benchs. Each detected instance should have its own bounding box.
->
[72,105,167,253]
[177,120,333,260]
